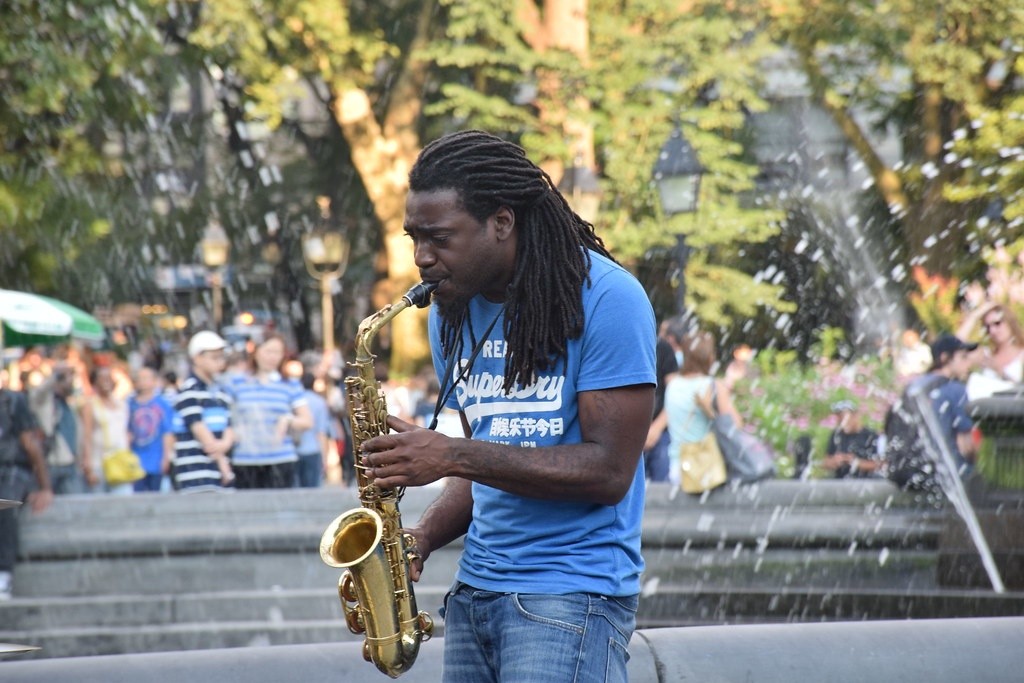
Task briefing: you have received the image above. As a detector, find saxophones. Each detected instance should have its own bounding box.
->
[320,282,440,678]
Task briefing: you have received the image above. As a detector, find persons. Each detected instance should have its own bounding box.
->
[0,330,459,603]
[361,130,656,678]
[644,300,1024,495]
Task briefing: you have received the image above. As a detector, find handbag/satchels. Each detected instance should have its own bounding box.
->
[104,451,147,485]
[0,391,35,469]
[679,431,729,505]
[709,376,780,487]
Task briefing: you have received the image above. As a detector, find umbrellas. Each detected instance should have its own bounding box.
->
[0,288,105,347]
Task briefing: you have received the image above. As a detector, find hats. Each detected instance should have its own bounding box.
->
[930,335,979,361]
[187,330,229,358]
[829,399,859,414]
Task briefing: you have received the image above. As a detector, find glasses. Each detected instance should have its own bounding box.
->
[987,319,1005,329]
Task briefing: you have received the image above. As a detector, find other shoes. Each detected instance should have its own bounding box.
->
[0,571,14,602]
